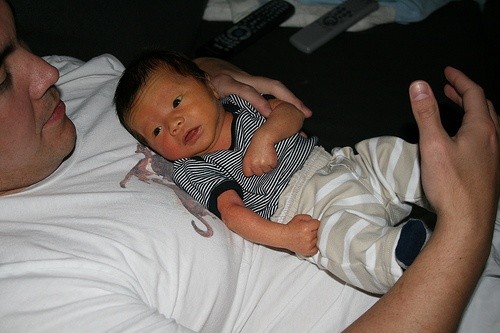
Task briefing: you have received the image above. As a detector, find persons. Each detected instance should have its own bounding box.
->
[114,47,436,296]
[0,0,500,333]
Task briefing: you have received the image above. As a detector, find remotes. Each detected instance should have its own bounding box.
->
[195,0,295,62]
[289,0,380,54]
